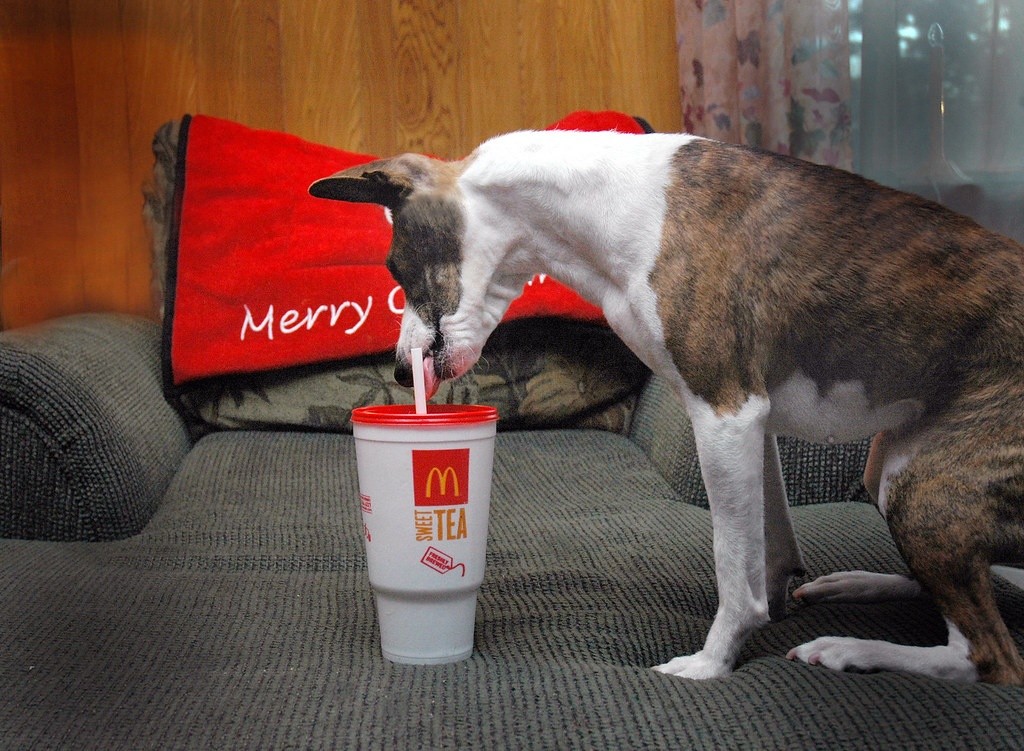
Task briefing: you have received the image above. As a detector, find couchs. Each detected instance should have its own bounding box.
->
[0,112,1024,751]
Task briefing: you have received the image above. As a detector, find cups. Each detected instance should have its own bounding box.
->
[350,404,500,666]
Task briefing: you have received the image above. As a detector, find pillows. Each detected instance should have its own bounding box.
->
[141,114,659,431]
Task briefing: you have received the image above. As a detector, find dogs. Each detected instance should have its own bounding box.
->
[307,127,1024,686]
